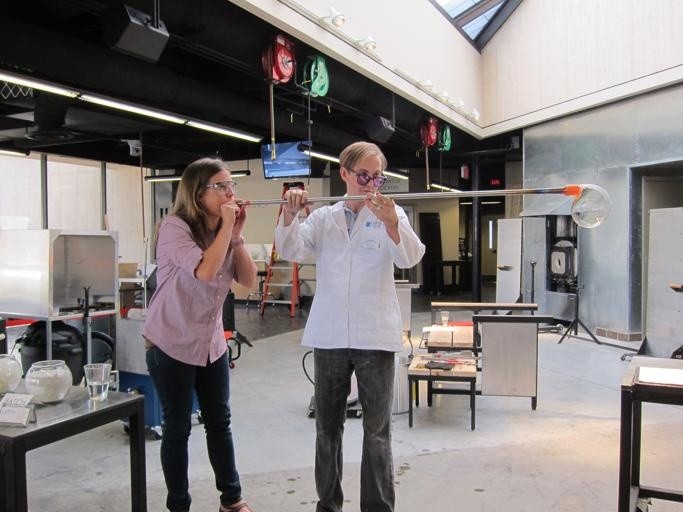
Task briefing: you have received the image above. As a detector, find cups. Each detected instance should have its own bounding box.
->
[440,311,449,327]
[84,362,119,402]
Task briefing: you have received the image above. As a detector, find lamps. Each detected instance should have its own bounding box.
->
[293,145,342,169]
[0,71,78,100]
[429,179,466,198]
[78,91,187,127]
[143,169,250,183]
[186,117,263,149]
[381,168,413,184]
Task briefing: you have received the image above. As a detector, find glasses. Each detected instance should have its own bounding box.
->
[204,180,237,192]
[348,168,388,188]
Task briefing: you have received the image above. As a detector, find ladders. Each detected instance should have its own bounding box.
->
[260,182,317,319]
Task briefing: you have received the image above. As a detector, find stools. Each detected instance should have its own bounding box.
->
[244,268,276,317]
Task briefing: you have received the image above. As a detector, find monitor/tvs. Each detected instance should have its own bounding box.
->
[260,139,313,179]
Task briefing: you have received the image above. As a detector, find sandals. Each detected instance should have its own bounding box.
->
[218,497,255,512]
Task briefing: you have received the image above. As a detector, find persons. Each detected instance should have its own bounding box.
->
[274,141,427,511]
[143,159,256,511]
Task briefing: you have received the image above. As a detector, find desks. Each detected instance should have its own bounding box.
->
[619,356,683,512]
[408,353,479,432]
[0,379,148,512]
[425,324,475,350]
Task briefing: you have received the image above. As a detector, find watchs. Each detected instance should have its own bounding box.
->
[232,236,244,248]
